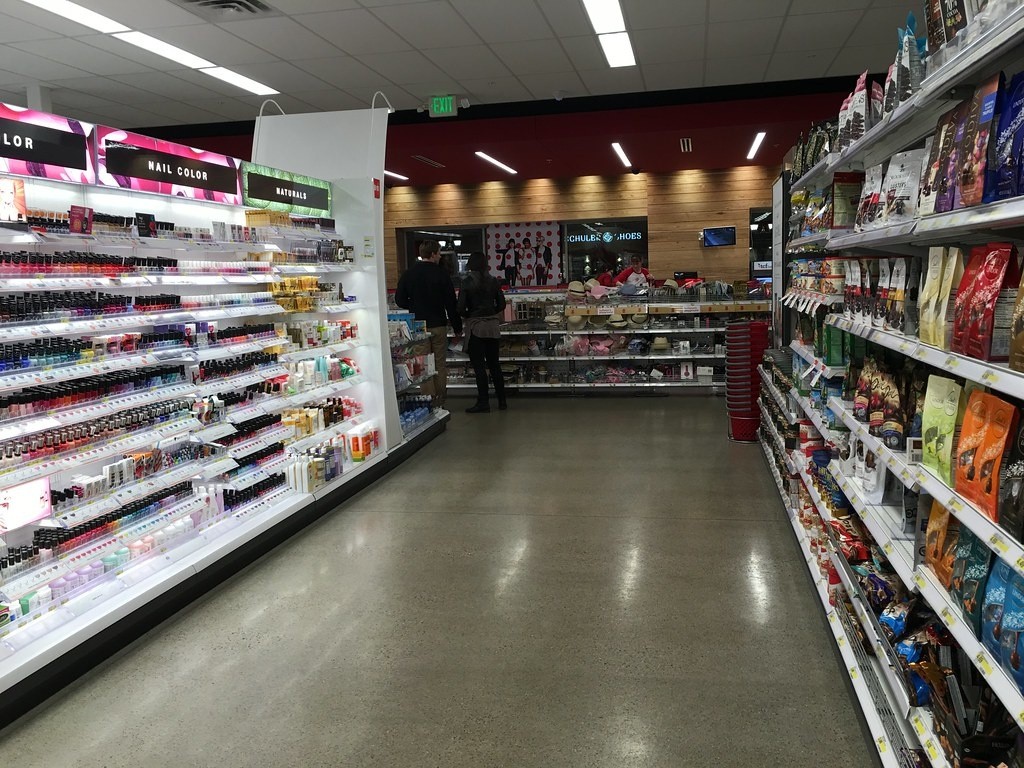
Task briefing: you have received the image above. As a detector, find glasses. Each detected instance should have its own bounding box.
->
[537,240,541,242]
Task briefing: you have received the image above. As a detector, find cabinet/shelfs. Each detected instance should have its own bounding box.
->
[648,302,773,397]
[564,304,648,395]
[0,101,389,732]
[390,335,451,471]
[445,326,564,397]
[755,1,1024,768]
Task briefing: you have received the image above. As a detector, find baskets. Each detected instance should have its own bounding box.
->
[725,319,769,443]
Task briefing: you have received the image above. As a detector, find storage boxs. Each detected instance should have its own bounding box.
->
[724,319,768,441]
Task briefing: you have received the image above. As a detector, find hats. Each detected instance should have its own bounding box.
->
[651,337,671,351]
[562,276,679,328]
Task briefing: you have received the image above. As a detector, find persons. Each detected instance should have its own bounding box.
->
[596,254,654,287]
[395,240,463,409]
[447,251,507,413]
[496,236,552,287]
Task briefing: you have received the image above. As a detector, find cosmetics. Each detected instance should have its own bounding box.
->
[399,394,432,430]
[0,206,378,638]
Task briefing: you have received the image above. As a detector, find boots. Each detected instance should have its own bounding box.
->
[465,394,491,412]
[496,391,507,410]
